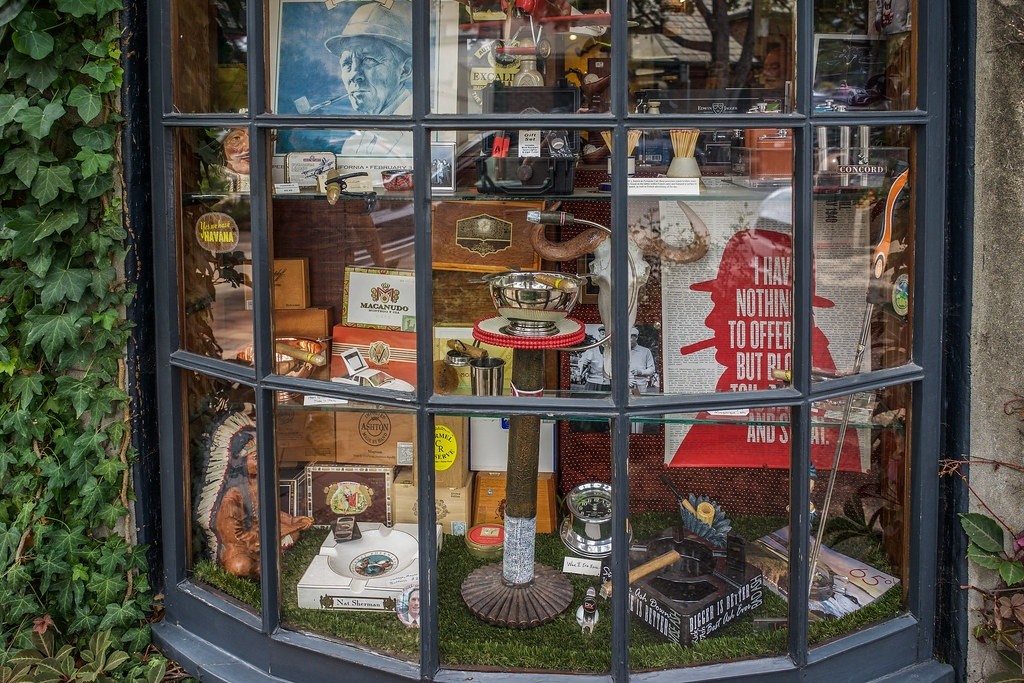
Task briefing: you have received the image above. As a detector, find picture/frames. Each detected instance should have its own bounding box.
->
[261,0,470,197]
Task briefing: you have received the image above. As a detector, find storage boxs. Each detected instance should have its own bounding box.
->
[475,85,581,195]
[217,201,559,539]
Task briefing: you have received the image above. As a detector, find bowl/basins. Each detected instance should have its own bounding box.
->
[482,271,582,337]
[667,157,701,178]
[381,169,414,191]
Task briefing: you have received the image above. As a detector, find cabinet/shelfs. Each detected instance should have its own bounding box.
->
[186,190,906,437]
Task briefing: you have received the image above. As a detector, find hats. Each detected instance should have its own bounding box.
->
[323,2,412,57]
[597,326,605,331]
[690,227,834,309]
[630,327,639,335]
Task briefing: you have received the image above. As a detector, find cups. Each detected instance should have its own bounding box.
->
[469,356,506,396]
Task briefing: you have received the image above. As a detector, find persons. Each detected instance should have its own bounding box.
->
[431,159,452,184]
[402,588,422,625]
[197,128,251,174]
[760,47,787,91]
[578,326,656,434]
[194,409,315,578]
[325,2,413,157]
